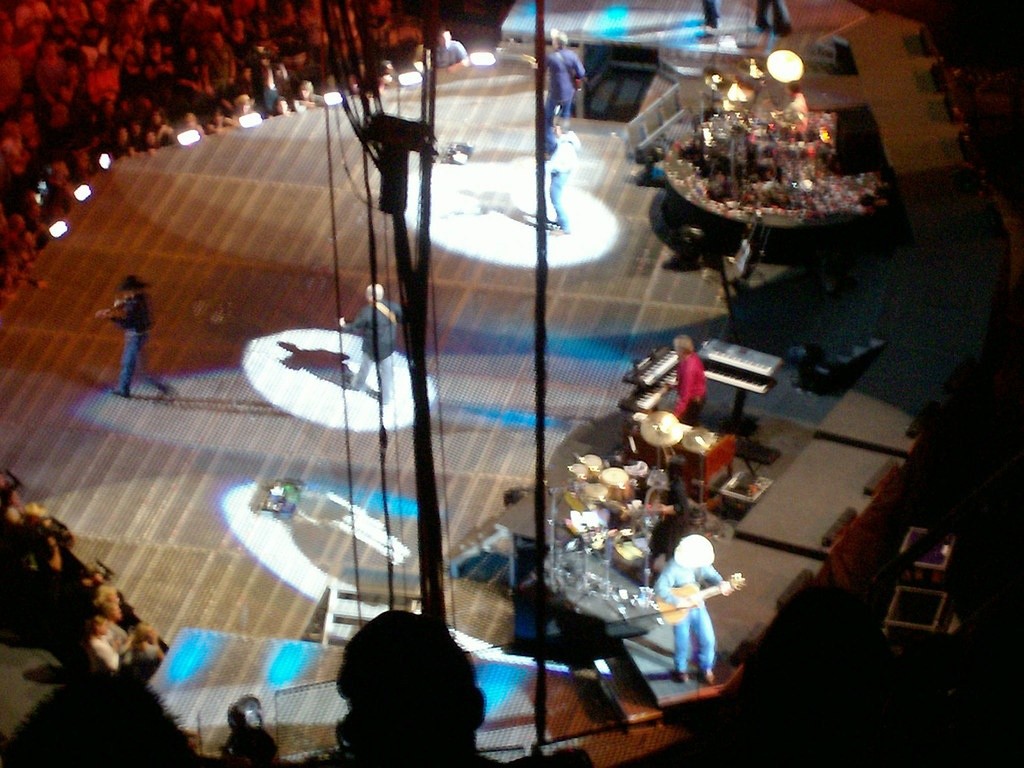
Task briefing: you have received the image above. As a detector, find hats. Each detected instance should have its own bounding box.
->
[673,534,715,568]
[116,275,147,290]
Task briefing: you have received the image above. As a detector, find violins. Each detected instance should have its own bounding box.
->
[96,295,132,318]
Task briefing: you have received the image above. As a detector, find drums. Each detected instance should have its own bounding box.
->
[567,453,650,506]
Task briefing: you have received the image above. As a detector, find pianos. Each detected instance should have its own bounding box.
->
[624,344,681,387]
[618,366,681,410]
[692,339,783,395]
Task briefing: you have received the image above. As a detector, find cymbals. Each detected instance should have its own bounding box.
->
[640,411,715,455]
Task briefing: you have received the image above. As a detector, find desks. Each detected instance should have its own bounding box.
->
[496,411,633,663]
[665,141,887,231]
[618,411,736,503]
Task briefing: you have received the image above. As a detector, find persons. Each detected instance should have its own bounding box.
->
[0,0,425,290]
[666,334,708,426]
[220,696,279,768]
[547,116,581,235]
[326,609,594,768]
[436,29,467,67]
[774,83,809,124]
[544,33,586,161]
[755,0,792,34]
[0,469,220,768]
[339,283,403,392]
[95,275,169,398]
[610,421,641,469]
[649,462,689,561]
[655,534,725,684]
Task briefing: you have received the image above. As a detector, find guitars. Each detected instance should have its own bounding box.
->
[656,573,747,625]
[522,51,536,65]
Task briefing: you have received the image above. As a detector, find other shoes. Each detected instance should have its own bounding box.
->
[679,672,689,682]
[703,670,715,684]
[113,390,129,397]
[158,385,168,399]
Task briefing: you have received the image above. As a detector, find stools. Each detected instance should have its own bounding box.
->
[735,440,782,476]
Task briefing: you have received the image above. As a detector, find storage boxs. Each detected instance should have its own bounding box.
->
[720,471,774,520]
